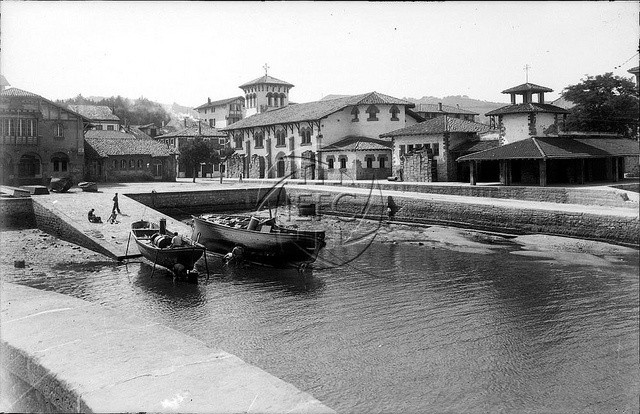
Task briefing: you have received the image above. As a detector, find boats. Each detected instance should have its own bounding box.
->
[130,220,206,270]
[191,213,325,264]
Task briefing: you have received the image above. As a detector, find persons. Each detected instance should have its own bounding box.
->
[87,208,102,222]
[108,210,118,224]
[171,231,182,247]
[113,192,120,212]
